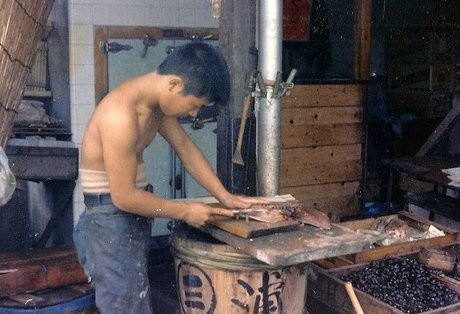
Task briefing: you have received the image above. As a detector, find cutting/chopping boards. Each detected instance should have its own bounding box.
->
[201,203,299,239]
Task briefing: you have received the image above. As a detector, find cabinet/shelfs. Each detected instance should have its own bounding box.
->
[280,79,368,218]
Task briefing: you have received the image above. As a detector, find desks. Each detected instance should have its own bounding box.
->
[380,154,460,221]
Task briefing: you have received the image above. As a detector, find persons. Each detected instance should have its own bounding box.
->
[72,40,273,314]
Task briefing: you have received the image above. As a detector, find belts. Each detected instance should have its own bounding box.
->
[83,184,153,205]
